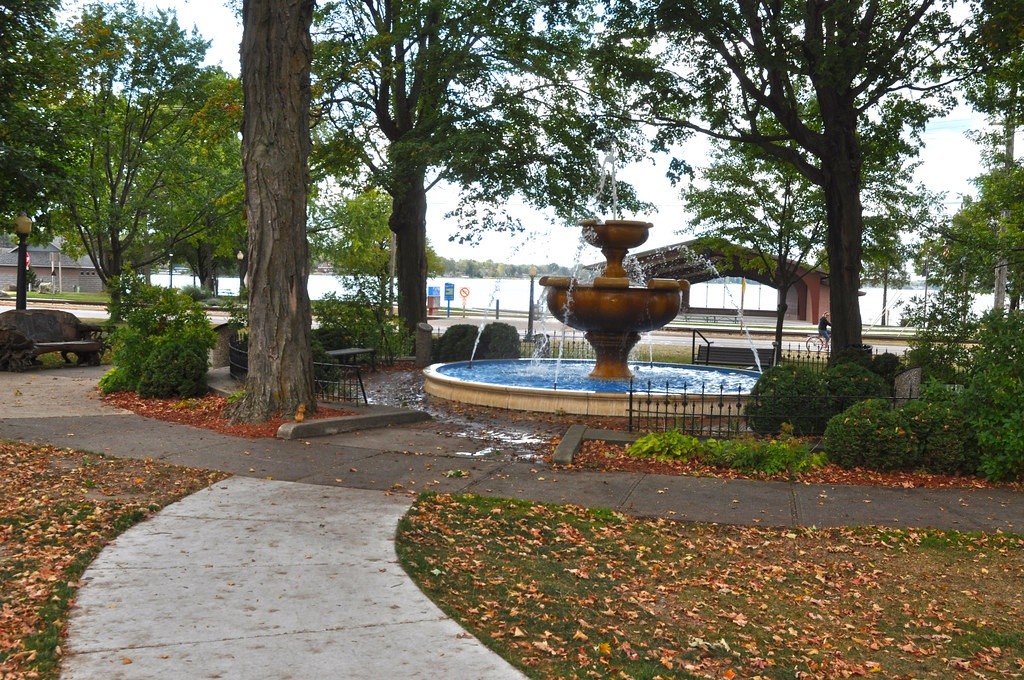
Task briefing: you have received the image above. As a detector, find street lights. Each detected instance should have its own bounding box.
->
[15,209,32,311]
[523,264,537,342]
[237,250,244,303]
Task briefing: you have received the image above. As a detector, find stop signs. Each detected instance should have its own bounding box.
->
[26,252,30,266]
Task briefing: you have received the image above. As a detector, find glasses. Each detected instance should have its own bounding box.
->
[826,315,829,316]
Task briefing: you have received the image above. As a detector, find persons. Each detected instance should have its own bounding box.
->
[818,312,831,348]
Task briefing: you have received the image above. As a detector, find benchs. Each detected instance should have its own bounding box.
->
[696,344,776,373]
[0,309,112,373]
[329,348,377,376]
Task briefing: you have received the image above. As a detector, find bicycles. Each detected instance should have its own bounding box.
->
[806,332,831,354]
